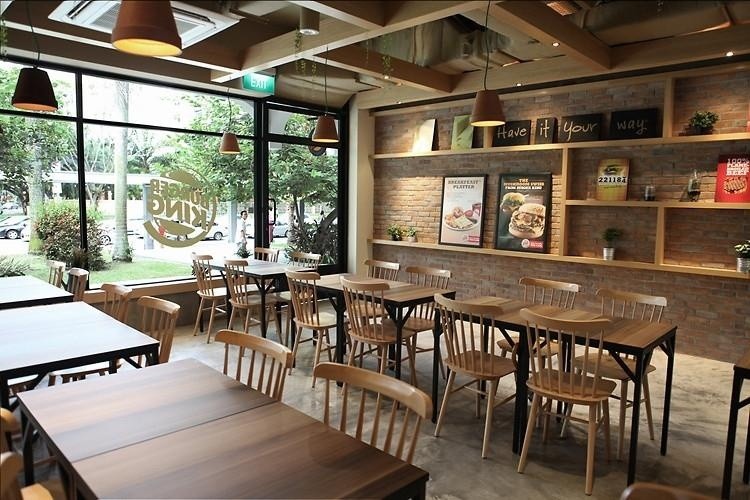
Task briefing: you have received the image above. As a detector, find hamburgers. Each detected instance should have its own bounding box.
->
[500,192,525,213]
[507,203,545,239]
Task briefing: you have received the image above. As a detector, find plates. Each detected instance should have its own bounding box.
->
[442,212,481,232]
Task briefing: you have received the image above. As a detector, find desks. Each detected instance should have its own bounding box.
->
[721,349,750,500]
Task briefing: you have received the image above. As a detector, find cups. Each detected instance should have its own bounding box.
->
[645,185,656,200]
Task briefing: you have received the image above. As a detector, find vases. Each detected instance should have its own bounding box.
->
[737,258,750,274]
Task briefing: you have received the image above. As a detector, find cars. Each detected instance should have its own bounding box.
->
[247,218,294,242]
[0,208,36,243]
[92,215,229,248]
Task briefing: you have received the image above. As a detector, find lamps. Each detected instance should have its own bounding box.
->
[312,48,340,142]
[220,70,239,155]
[470,0,506,129]
[11,1,58,112]
[111,0,183,58]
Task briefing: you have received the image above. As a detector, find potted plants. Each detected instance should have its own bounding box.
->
[688,110,719,135]
[601,228,622,260]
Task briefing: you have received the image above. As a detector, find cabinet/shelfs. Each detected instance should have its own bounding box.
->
[367,61,750,279]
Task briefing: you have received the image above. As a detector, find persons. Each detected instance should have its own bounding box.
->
[320,211,326,225]
[269,207,274,242]
[235,211,248,254]
[159,226,166,248]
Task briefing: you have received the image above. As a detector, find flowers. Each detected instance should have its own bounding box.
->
[388,223,415,241]
[733,244,750,258]
